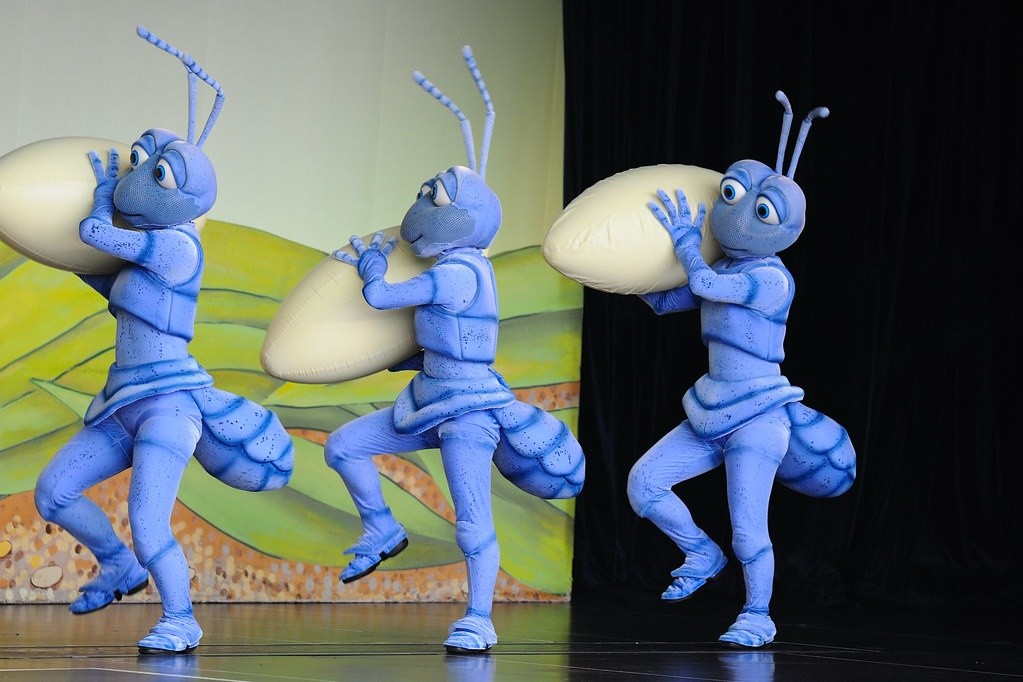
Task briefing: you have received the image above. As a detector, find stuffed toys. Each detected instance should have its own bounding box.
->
[34,26,296,653]
[326,45,585,653]
[626,90,856,647]
